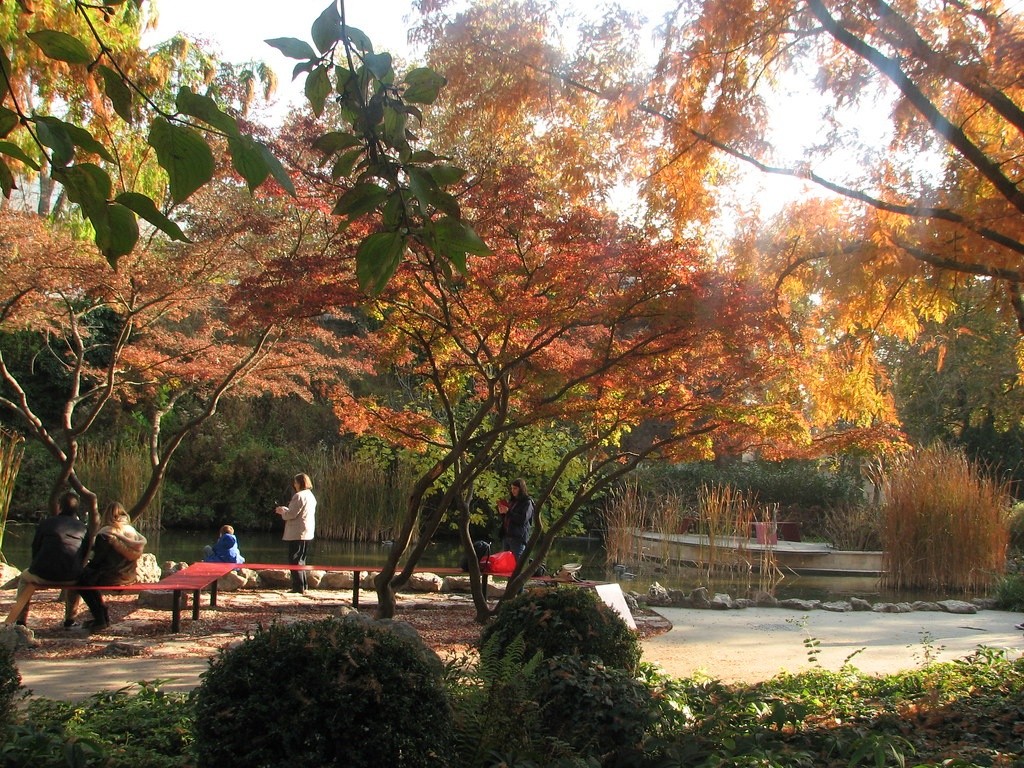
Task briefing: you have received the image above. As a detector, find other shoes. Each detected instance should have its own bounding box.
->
[287,590,304,595]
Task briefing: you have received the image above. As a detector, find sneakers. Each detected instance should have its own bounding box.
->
[63,619,82,631]
[16,620,27,629]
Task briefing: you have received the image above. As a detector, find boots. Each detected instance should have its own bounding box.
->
[81,606,112,631]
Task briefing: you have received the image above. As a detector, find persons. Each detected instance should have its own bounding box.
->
[275,473,317,593]
[202,525,245,572]
[76,501,147,629]
[16,493,89,630]
[499,478,535,597]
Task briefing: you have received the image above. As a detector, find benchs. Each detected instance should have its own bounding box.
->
[6,561,638,635]
[680,517,802,544]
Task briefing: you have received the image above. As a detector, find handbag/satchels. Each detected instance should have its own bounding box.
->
[479,550,517,573]
[551,563,584,583]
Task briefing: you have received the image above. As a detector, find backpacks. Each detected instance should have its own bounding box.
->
[460,539,492,573]
